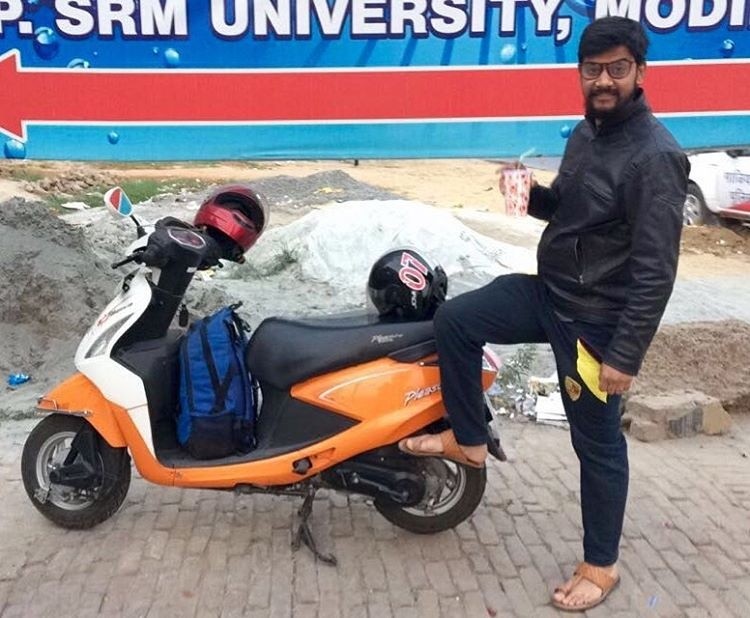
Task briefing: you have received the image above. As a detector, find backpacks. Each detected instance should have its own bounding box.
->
[176,301,258,459]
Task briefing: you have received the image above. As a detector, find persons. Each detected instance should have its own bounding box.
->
[398,12,692,610]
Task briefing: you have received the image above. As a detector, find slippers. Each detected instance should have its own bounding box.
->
[552,562,620,612]
[397,427,484,470]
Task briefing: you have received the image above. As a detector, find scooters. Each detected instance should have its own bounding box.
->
[20,185,503,567]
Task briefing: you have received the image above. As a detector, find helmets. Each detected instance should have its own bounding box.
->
[369,249,443,320]
[195,185,269,254]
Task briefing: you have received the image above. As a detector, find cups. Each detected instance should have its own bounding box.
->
[502,169,533,216]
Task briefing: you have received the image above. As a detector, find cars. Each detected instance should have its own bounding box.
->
[679,149,750,228]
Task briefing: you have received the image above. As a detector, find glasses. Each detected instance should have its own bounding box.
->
[578,60,635,80]
